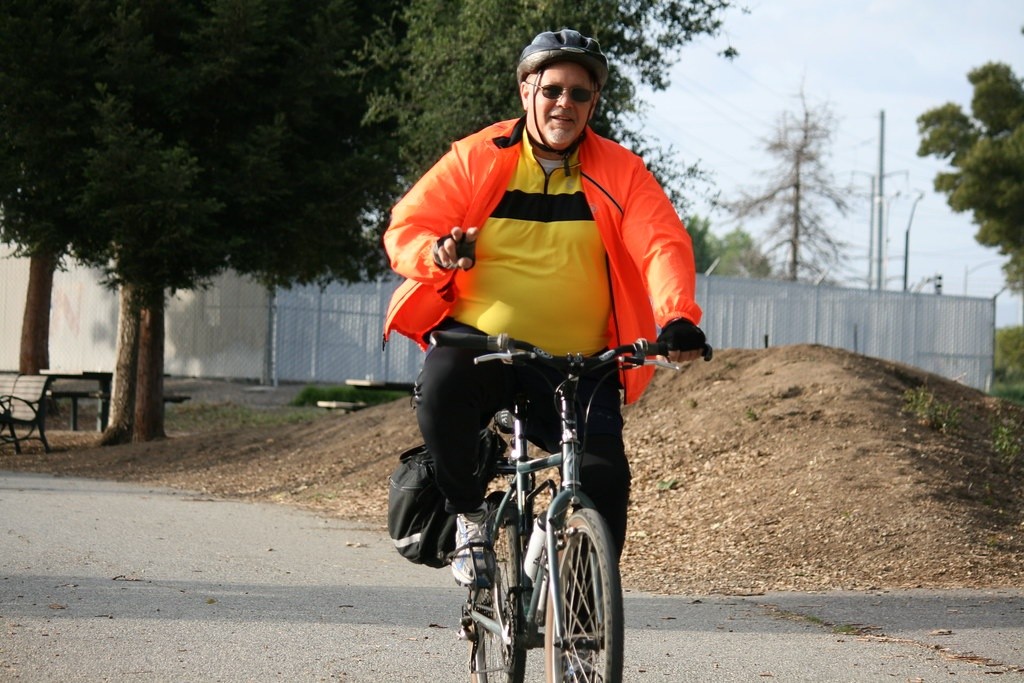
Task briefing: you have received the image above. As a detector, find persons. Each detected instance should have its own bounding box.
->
[380,28,706,683]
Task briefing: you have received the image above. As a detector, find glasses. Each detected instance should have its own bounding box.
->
[523,81,599,102]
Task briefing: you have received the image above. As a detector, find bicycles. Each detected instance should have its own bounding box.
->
[419,328,716,683]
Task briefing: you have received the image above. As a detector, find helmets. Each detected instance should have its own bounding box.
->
[516,30,609,93]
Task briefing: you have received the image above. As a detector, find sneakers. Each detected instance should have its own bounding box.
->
[559,647,595,683]
[452,507,490,583]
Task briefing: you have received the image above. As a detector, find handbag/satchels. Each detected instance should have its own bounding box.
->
[387,443,457,569]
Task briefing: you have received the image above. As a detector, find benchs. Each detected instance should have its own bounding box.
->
[0,371,54,452]
[316,402,369,415]
[45,389,191,431]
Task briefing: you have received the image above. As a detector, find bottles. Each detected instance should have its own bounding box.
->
[522,510,549,582]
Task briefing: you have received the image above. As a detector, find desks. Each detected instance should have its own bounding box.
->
[43,368,172,430]
[347,377,416,404]
